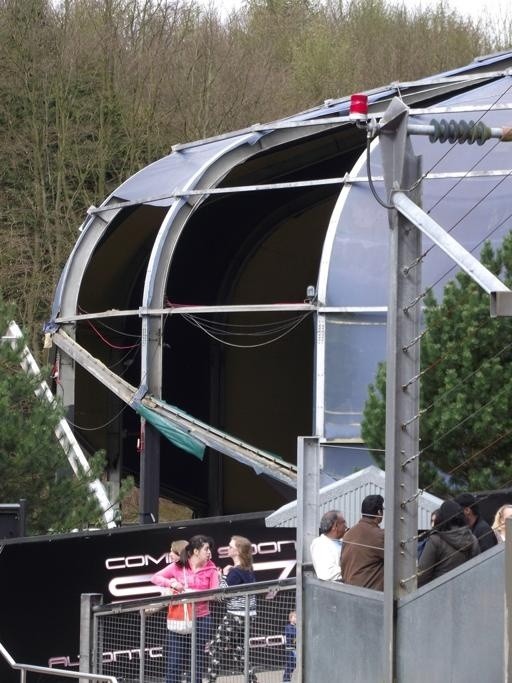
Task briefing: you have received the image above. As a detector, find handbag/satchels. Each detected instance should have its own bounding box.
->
[165,591,195,635]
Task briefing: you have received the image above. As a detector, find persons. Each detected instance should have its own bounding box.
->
[340,494,384,591]
[310,510,349,583]
[151,535,220,683]
[144,540,189,617]
[283,609,297,682]
[418,494,512,588]
[206,536,257,683]
